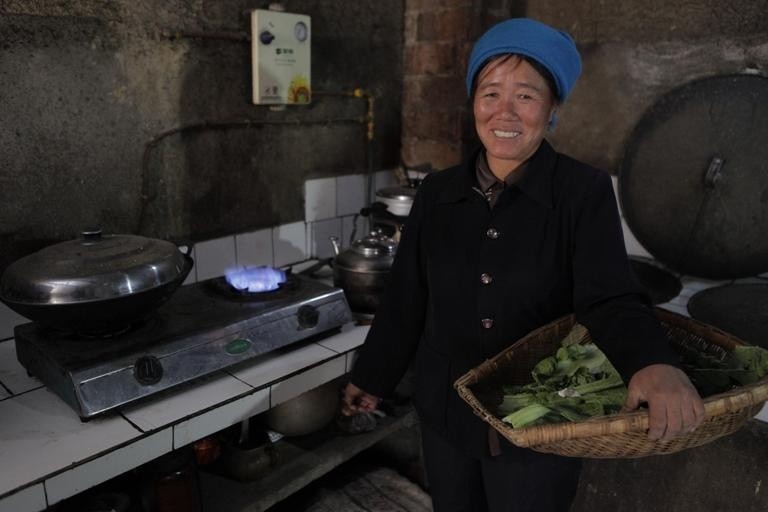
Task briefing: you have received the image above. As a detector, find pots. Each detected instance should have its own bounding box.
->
[374,176,423,225]
[0,224,196,336]
[685,278,767,352]
[626,255,683,308]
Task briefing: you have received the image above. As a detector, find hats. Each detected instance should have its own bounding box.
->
[465,17,584,102]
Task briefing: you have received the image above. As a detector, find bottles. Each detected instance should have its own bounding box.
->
[154,456,204,512]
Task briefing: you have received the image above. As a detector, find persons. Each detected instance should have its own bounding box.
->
[339,17,707,510]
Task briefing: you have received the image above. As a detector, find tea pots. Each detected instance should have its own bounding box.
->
[326,204,402,315]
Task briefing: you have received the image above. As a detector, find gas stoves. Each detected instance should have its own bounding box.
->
[13,264,354,425]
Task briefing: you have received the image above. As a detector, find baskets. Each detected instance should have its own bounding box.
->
[453,295,766,460]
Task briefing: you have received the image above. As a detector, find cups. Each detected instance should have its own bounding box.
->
[222,439,281,483]
